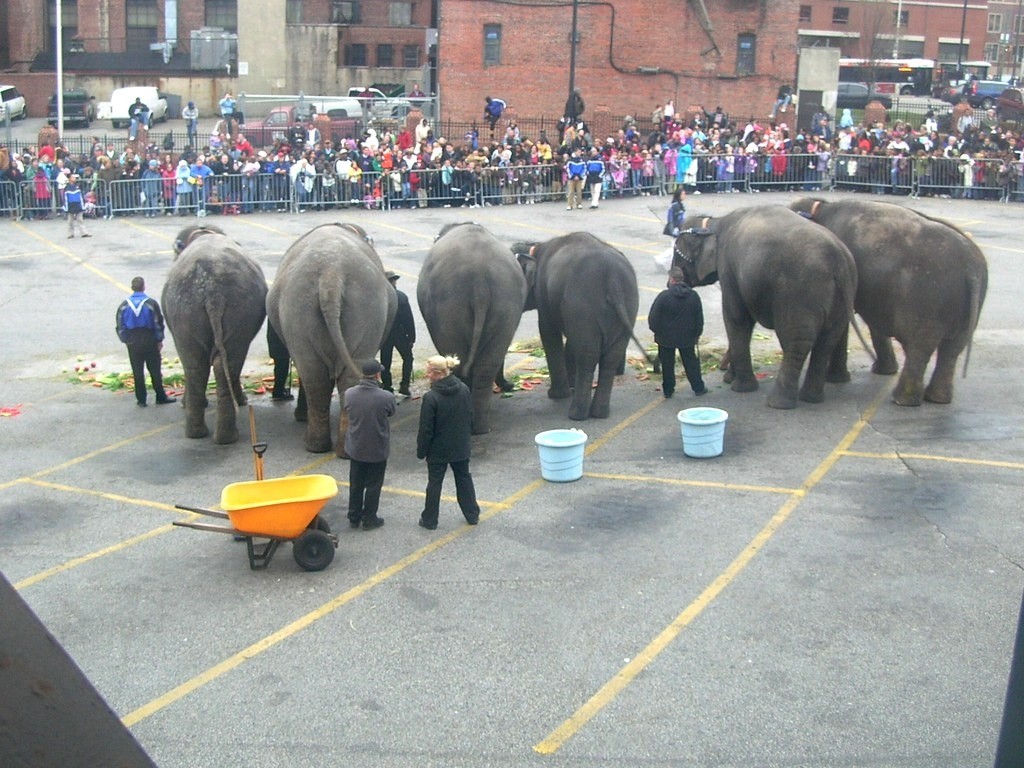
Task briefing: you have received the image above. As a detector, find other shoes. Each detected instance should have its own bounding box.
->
[590,206,598,209]
[349,518,360,528]
[466,515,478,525]
[442,201,536,209]
[129,136,136,140]
[137,400,146,407]
[154,397,176,404]
[399,387,411,396]
[577,204,583,209]
[82,234,93,237]
[419,517,437,530]
[567,205,572,210]
[663,388,675,398]
[144,125,149,130]
[635,188,760,196]
[362,518,384,530]
[695,386,708,396]
[273,393,294,400]
[68,235,73,239]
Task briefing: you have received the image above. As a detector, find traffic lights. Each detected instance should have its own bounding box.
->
[1004,46,1011,56]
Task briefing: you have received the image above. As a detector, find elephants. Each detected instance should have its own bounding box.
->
[161,224,270,444]
[417,221,529,436]
[667,204,858,409]
[785,197,989,407]
[509,231,639,421]
[265,222,398,459]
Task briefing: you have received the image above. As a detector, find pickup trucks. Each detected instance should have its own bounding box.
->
[46,88,97,129]
[309,87,413,119]
[237,106,361,148]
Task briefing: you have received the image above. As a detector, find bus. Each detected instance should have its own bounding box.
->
[839,56,933,97]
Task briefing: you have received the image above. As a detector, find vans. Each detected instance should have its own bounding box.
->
[108,86,169,129]
[0,84,28,124]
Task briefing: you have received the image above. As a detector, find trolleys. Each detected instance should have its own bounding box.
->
[172,474,339,572]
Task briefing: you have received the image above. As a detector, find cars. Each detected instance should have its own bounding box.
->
[940,84,966,105]
[836,82,893,110]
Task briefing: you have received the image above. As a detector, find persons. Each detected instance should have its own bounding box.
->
[0,75,1023,222]
[648,266,708,399]
[267,317,294,400]
[63,173,92,238]
[662,188,686,237]
[380,271,415,399]
[344,359,397,531]
[416,353,481,529]
[116,277,177,407]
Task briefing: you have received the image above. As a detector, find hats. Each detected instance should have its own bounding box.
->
[361,358,385,375]
[385,270,400,280]
[668,267,684,279]
[148,159,157,167]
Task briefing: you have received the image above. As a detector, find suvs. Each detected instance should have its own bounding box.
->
[962,80,1011,110]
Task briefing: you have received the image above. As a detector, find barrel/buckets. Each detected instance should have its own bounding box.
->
[677,407,728,458]
[535,428,588,483]
